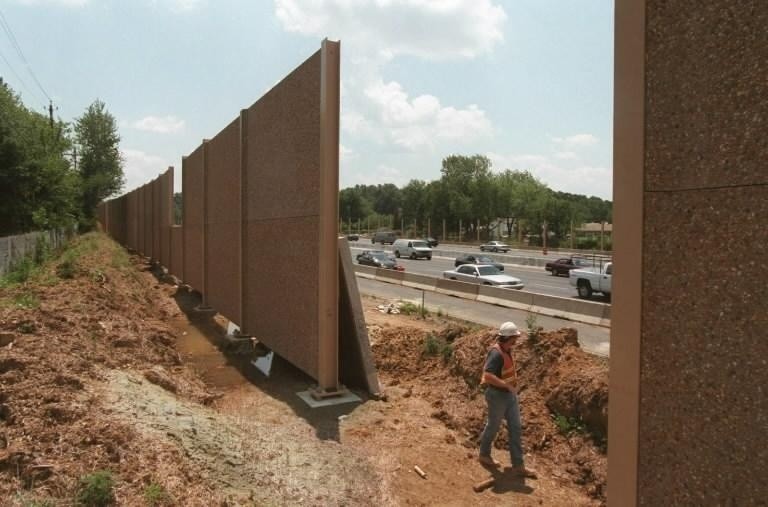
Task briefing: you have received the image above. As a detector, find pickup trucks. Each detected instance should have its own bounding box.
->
[569,261,612,298]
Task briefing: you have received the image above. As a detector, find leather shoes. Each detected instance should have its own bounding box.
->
[478,454,501,467]
[512,465,537,477]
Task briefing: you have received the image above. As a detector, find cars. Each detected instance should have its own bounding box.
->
[443,238,525,289]
[545,256,592,276]
[359,254,397,268]
[421,237,437,246]
[343,233,359,241]
[356,250,390,259]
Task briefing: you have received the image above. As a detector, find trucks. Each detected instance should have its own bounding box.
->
[393,237,432,259]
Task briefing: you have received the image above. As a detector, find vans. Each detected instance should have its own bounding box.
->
[371,232,394,244]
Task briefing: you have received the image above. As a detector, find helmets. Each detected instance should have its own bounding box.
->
[498,322,520,336]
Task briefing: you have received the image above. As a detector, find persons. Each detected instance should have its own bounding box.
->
[478,322,537,476]
